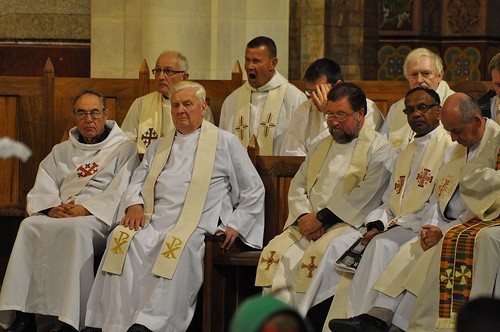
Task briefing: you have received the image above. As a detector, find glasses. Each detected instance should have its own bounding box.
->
[304,85,334,97]
[74,110,104,119]
[403,103,437,114]
[324,111,356,121]
[152,68,185,77]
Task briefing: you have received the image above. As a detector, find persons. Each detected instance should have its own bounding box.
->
[120,50,214,154]
[218,36,308,157]
[85,81,265,332]
[230,48,500,332]
[0,89,142,332]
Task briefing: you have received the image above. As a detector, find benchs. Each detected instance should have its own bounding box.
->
[202,134,306,332]
[0,56,495,305]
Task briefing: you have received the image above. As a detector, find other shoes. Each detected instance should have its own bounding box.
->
[3,312,37,332]
[329,314,389,332]
